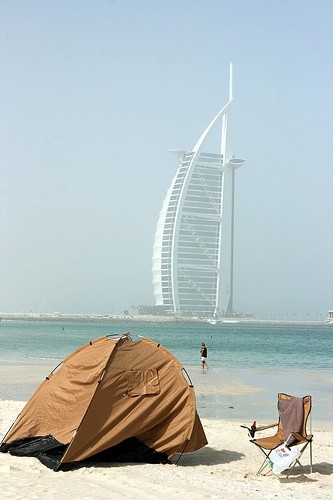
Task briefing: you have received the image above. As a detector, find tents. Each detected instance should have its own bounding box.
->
[0,334,209,472]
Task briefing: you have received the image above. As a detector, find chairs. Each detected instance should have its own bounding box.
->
[241,390,314,480]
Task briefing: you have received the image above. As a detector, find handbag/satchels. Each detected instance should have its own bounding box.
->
[267,441,300,476]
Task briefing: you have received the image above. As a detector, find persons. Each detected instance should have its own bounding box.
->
[200,343,208,369]
